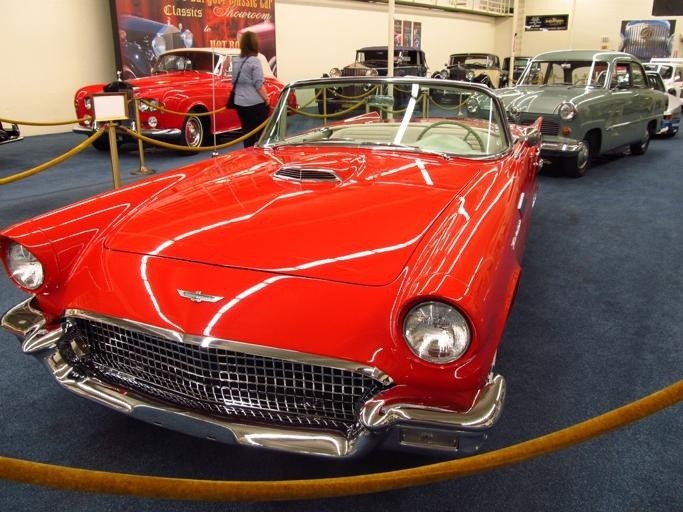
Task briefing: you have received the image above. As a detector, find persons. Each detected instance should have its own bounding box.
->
[230,31,272,149]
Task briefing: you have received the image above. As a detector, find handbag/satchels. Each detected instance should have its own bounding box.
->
[225,88,236,110]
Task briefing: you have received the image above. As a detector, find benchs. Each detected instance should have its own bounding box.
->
[328,123,496,155]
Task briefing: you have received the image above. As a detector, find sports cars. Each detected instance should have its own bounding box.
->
[0,76,544,467]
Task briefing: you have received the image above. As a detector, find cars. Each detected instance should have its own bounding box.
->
[593,56,682,137]
[117,12,199,78]
[72,41,299,153]
[311,41,431,119]
[428,50,505,103]
[467,49,670,174]
[499,54,542,88]
